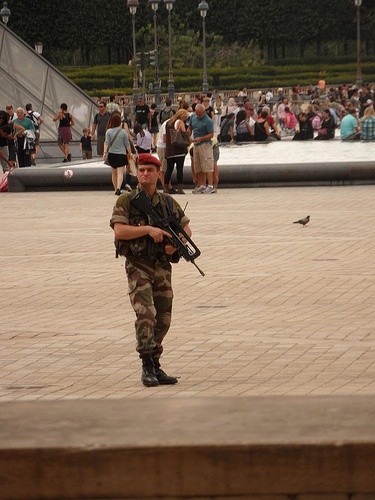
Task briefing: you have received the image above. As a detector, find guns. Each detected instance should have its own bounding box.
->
[130,190,206,277]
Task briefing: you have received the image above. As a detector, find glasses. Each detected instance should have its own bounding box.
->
[97,106,104,108]
[138,100,143,104]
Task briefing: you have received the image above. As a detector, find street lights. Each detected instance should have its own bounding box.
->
[150,0,161,104]
[165,0,176,96]
[352,0,363,80]
[126,0,140,103]
[197,0,210,95]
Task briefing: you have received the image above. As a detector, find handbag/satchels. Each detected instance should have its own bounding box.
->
[214,108,221,114]
[35,131,40,145]
[312,116,321,129]
[0,166,12,192]
[217,134,222,142]
[22,130,35,150]
[103,152,110,166]
[171,129,191,146]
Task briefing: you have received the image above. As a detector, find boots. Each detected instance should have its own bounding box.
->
[175,182,185,194]
[164,182,176,193]
[152,354,178,385]
[141,350,159,386]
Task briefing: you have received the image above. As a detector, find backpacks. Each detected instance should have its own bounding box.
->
[25,111,37,130]
[160,111,169,125]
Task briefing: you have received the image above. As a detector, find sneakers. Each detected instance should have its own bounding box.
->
[201,185,214,194]
[192,185,206,194]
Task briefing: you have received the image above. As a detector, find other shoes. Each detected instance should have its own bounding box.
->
[67,153,71,162]
[114,187,122,195]
[210,188,217,193]
[62,158,69,162]
[151,145,157,153]
[125,183,132,192]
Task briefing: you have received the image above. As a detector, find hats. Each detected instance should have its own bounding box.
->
[362,99,375,107]
[345,106,361,113]
[135,153,162,167]
[276,87,284,92]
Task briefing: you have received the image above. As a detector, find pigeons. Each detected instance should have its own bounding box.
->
[292,216,310,228]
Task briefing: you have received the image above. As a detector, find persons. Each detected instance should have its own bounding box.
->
[110,153,192,387]
[0,103,75,173]
[80,80,375,194]
